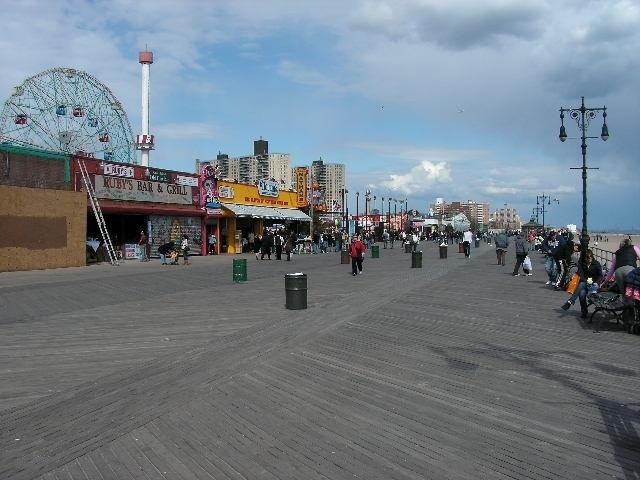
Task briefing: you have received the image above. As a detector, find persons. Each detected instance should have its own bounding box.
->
[561,248,603,317]
[349,231,375,276]
[463,228,492,258]
[139,231,151,261]
[603,238,640,334]
[495,230,532,276]
[241,230,301,261]
[305,230,347,253]
[529,229,579,290]
[382,230,420,251]
[208,233,216,254]
[422,230,463,244]
[159,235,189,265]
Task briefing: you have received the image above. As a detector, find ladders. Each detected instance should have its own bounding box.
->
[78,159,120,267]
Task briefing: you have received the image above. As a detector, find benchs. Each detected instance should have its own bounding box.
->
[587,291,634,324]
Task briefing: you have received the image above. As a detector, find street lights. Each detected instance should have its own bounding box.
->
[374,197,409,230]
[558,96,609,249]
[531,192,550,227]
[345,191,359,233]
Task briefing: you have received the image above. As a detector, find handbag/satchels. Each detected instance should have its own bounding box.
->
[593,292,619,304]
[568,270,582,295]
[599,285,608,291]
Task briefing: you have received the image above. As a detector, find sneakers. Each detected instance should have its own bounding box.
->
[546,279,573,294]
[526,273,532,276]
[512,273,520,277]
[561,301,572,311]
[359,271,362,275]
[579,310,588,318]
[353,273,357,276]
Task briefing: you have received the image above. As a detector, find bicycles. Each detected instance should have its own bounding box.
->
[465,246,471,263]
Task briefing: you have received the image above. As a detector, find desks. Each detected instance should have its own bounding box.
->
[121,244,146,260]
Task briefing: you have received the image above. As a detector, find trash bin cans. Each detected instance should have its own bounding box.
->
[487,238,491,244]
[372,246,379,258]
[405,243,412,253]
[412,251,423,268]
[341,249,350,264]
[459,242,465,253]
[439,245,448,259]
[475,240,480,248]
[284,272,307,310]
[233,258,247,282]
